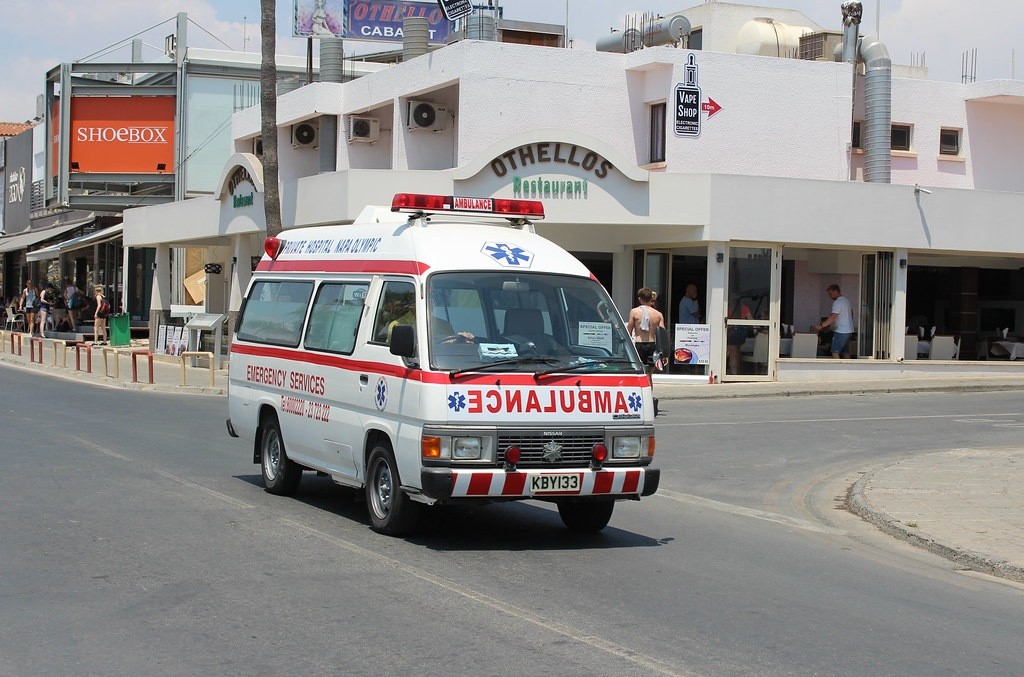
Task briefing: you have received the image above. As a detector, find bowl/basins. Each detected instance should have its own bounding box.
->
[674,348,692,363]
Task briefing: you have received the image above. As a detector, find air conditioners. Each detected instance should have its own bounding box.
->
[405,99,447,131]
[290,123,319,148]
[346,114,379,143]
[251,137,263,156]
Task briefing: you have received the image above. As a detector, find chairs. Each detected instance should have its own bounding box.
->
[975,336,1002,361]
[739,332,770,375]
[791,333,818,359]
[928,335,955,360]
[4,308,26,333]
[905,335,918,360]
[503,308,544,338]
[952,337,961,360]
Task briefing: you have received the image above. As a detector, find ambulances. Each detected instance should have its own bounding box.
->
[226,193,661,538]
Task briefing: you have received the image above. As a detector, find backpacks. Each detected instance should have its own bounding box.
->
[70,287,79,309]
[98,294,110,319]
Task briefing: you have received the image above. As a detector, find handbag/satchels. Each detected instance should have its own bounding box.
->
[32,298,40,308]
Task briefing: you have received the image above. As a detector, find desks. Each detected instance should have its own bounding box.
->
[741,338,792,357]
[991,340,1024,360]
[917,339,958,360]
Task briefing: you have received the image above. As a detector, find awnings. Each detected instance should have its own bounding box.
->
[24,222,123,263]
[0,218,96,254]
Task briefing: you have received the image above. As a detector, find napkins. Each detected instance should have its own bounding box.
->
[1003,328,1008,339]
[918,326,924,337]
[905,326,909,334]
[790,325,794,334]
[930,326,936,337]
[782,323,789,335]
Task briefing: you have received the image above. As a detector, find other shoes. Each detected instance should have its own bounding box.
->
[92,343,97,345]
[99,343,107,345]
[40,335,47,338]
[653,398,658,417]
[29,333,34,337]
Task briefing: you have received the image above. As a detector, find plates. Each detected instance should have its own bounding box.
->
[675,350,698,364]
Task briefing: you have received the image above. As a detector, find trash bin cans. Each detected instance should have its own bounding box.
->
[109,313,131,346]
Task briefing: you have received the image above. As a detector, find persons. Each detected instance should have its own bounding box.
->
[727,290,757,375]
[0,283,91,332]
[63,278,85,331]
[627,287,669,418]
[40,283,55,338]
[91,287,108,346]
[19,279,39,337]
[813,284,856,358]
[386,281,475,358]
[678,284,702,375]
